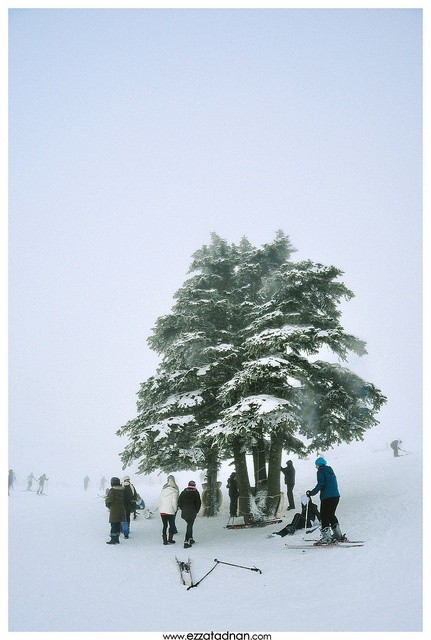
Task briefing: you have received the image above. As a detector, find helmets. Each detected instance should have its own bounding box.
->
[315,457,327,466]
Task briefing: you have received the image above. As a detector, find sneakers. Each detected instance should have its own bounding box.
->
[318,528,335,544]
[331,523,343,540]
[190,539,195,544]
[184,542,192,548]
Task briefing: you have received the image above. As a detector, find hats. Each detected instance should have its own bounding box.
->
[111,477,120,486]
[168,479,176,487]
[123,475,130,481]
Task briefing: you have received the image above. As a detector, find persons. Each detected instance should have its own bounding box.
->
[226,472,239,517]
[82,476,89,491]
[306,457,341,544]
[7,469,15,489]
[105,477,126,544]
[120,475,136,527]
[35,474,49,495]
[24,472,35,492]
[163,475,179,535]
[159,479,179,545]
[99,476,107,490]
[119,520,129,539]
[177,481,201,548]
[280,460,295,509]
[270,494,321,538]
[390,440,402,456]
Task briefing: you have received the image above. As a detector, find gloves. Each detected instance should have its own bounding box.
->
[306,490,313,497]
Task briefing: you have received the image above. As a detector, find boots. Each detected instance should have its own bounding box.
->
[163,535,168,545]
[169,532,175,543]
[106,534,120,544]
